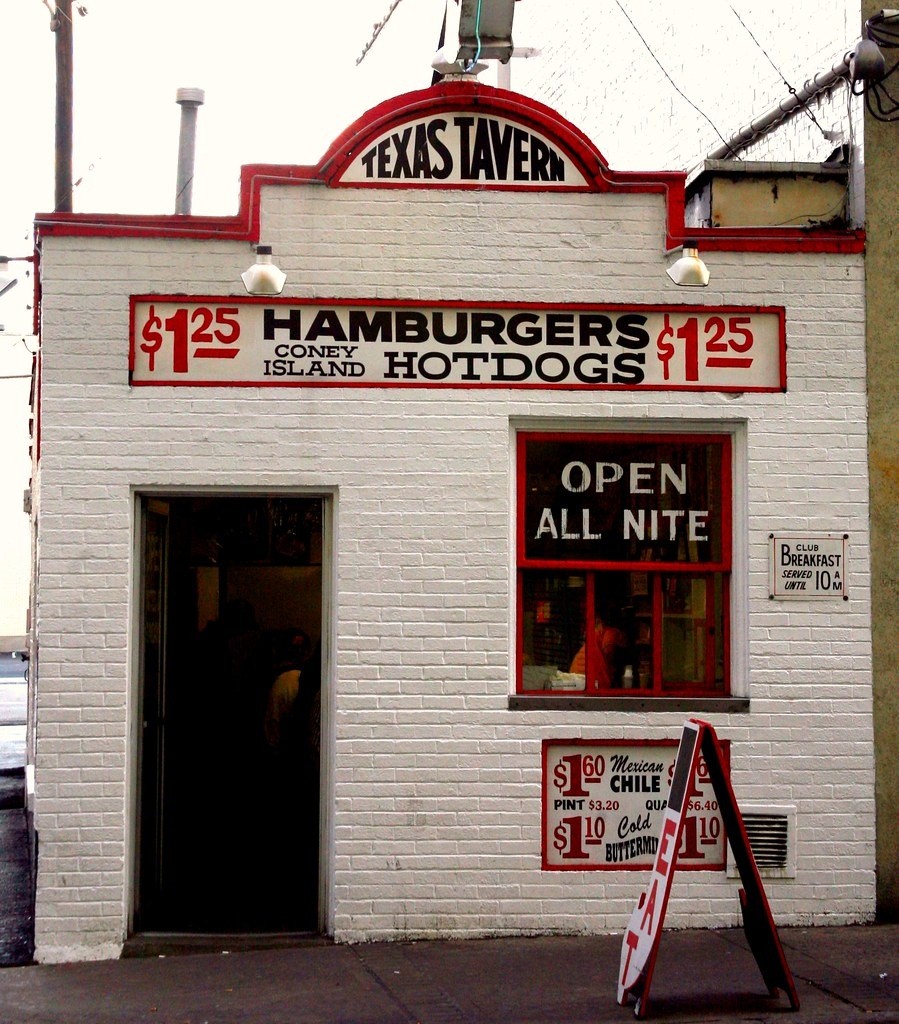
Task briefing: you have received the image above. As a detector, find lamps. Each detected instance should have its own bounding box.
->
[665,240,711,288]
[240,244,287,295]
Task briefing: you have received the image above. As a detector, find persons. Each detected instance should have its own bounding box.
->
[569,601,633,687]
[262,642,320,882]
